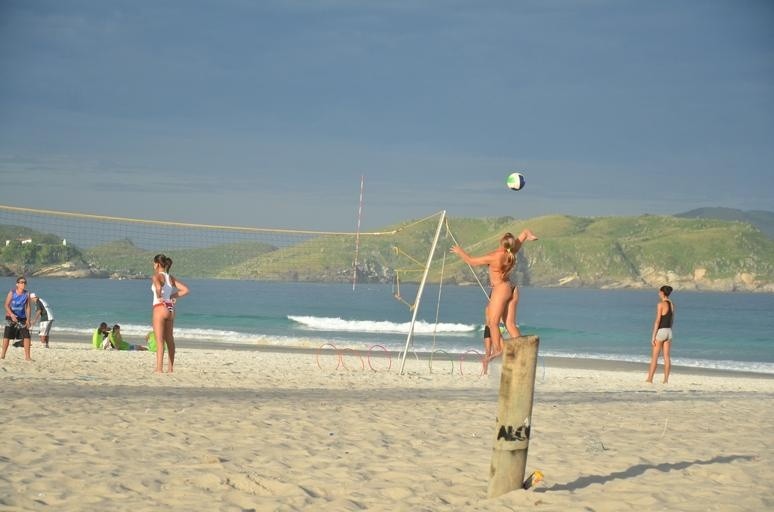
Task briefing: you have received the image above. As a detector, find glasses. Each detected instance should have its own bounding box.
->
[16,281,27,283]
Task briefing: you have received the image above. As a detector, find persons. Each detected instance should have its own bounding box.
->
[29,292,55,348]
[108,325,147,350]
[145,326,167,353]
[151,254,189,372]
[646,285,674,385]
[450,230,538,361]
[92,322,110,350]
[0,278,35,362]
[482,290,505,374]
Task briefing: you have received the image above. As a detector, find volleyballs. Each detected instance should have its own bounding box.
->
[507,172,525,192]
[498,322,506,334]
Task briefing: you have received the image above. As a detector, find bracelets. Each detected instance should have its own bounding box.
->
[11,314,15,318]
